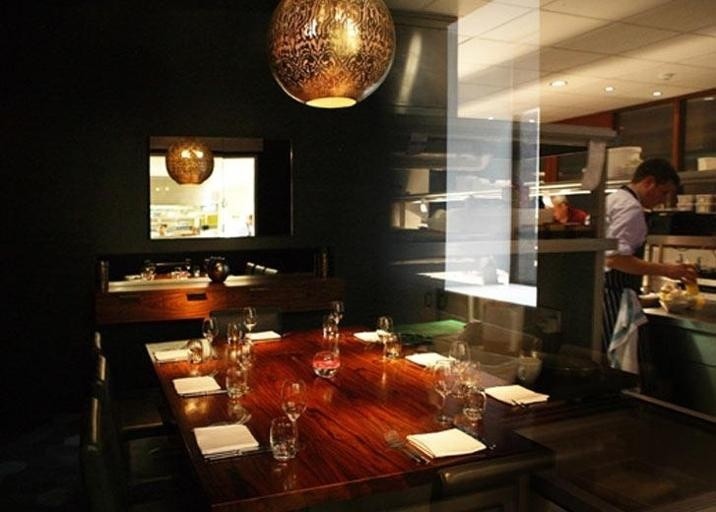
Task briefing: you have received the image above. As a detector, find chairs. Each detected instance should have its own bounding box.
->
[83,332,211,512]
[429,452,555,512]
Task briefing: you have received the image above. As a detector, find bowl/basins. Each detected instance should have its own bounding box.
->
[658,298,697,314]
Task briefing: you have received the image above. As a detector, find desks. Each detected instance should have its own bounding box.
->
[95,273,347,330]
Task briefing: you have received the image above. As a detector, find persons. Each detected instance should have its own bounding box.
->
[584,158,698,396]
[540,194,587,225]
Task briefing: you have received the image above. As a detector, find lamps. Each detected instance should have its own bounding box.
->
[269,1,397,110]
[165,138,214,185]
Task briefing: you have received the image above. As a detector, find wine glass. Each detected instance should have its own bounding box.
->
[281,379,308,451]
[377,315,393,364]
[202,306,257,360]
[330,299,344,339]
[430,340,481,424]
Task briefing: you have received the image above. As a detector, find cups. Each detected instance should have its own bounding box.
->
[323,319,336,338]
[226,365,249,399]
[388,332,402,359]
[518,357,543,384]
[270,417,297,461]
[463,384,488,422]
